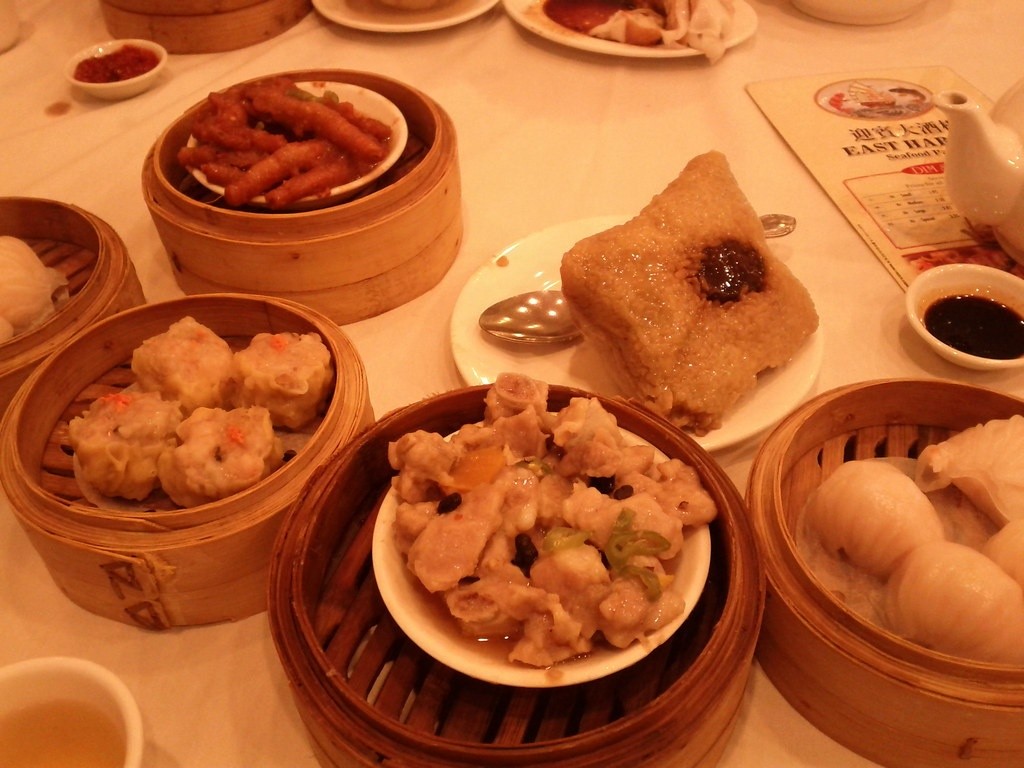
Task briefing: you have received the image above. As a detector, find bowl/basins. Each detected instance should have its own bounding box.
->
[0,657,144,768]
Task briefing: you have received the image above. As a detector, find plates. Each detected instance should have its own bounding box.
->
[64,37,169,100]
[903,263,1024,372]
[184,80,409,209]
[502,0,760,57]
[455,216,825,455]
[311,1,497,33]
[369,404,713,689]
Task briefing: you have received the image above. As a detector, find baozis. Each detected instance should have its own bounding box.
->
[815,413,1023,670]
[1,234,69,343]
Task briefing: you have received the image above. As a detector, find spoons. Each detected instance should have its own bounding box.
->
[479,213,798,342]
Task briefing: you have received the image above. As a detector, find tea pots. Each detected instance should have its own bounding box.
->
[933,64,1024,262]
